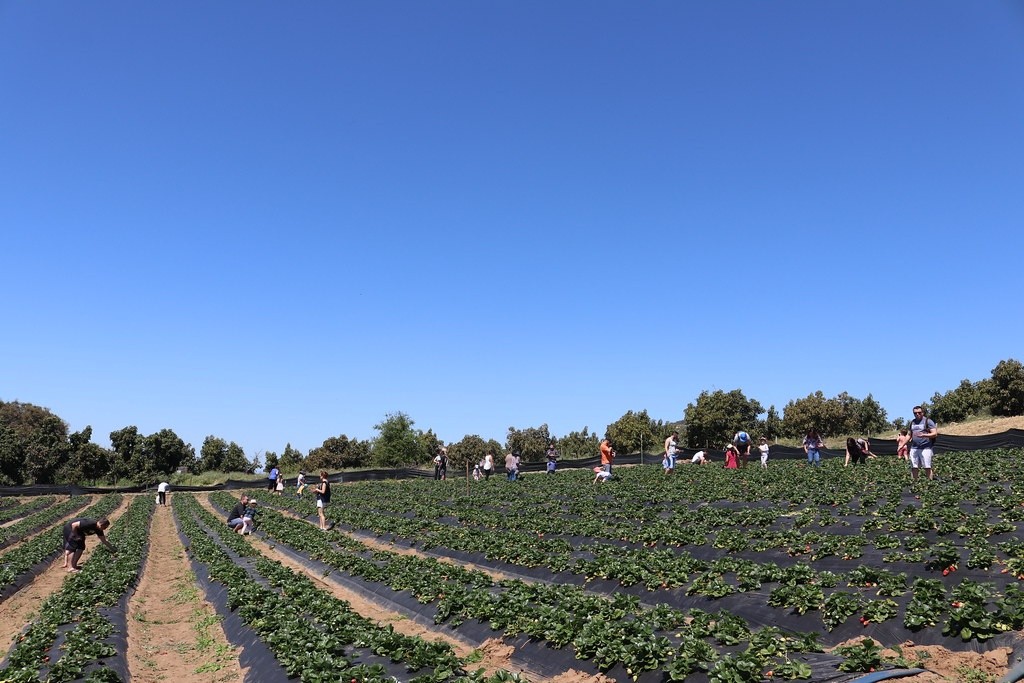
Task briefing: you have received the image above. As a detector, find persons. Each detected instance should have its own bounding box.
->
[723,431,769,469]
[662,430,681,474]
[297,484,308,499]
[593,436,614,484]
[473,450,495,481]
[227,494,261,535]
[803,427,823,466]
[300,472,306,486]
[297,470,304,489]
[545,445,559,474]
[158,481,170,507]
[692,448,710,466]
[844,437,868,467]
[316,470,329,530]
[897,405,937,481]
[268,466,279,492]
[276,474,284,495]
[434,449,447,480]
[504,450,521,481]
[63,517,117,573]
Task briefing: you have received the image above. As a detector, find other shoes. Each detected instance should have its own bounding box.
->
[68,565,83,573]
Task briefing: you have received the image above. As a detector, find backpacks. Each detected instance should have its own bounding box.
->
[910,418,936,443]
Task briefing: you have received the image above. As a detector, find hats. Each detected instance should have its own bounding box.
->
[740,433,747,442]
[249,499,257,504]
[726,444,733,449]
[515,451,520,455]
[759,437,767,441]
[549,445,554,449]
[594,466,601,473]
[475,465,478,468]
[299,469,306,474]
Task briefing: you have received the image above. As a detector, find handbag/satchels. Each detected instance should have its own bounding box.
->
[857,437,870,449]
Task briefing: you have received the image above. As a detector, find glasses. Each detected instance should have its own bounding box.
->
[914,411,922,415]
